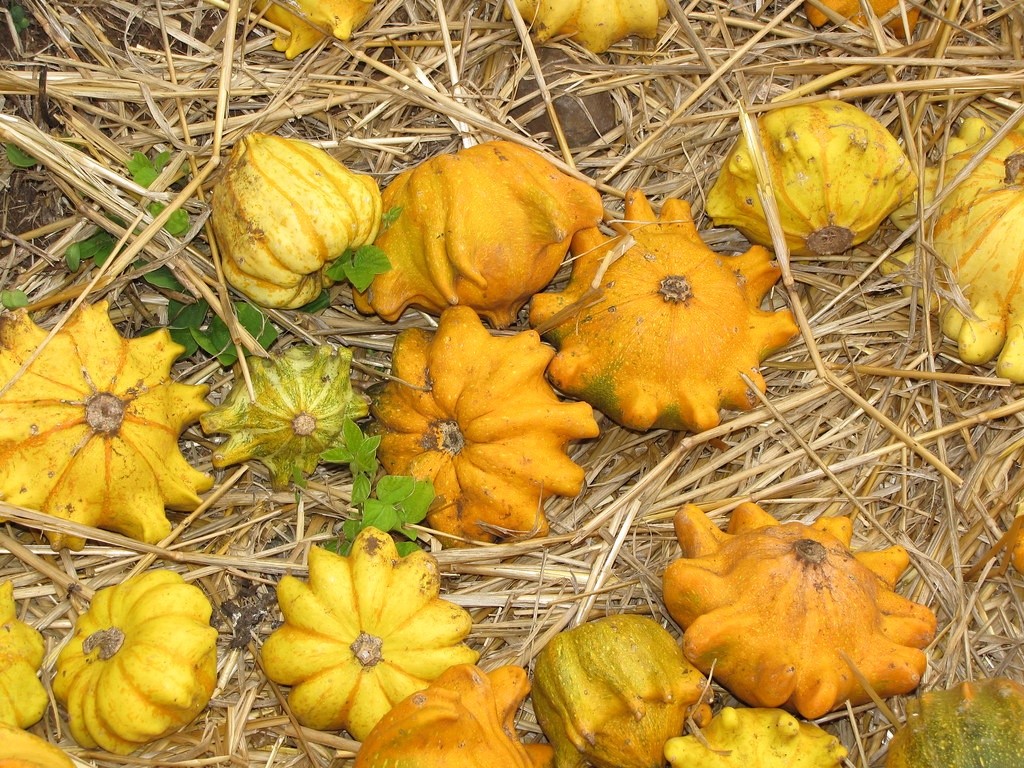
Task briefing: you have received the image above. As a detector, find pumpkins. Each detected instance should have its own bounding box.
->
[0,1,1024,768]
